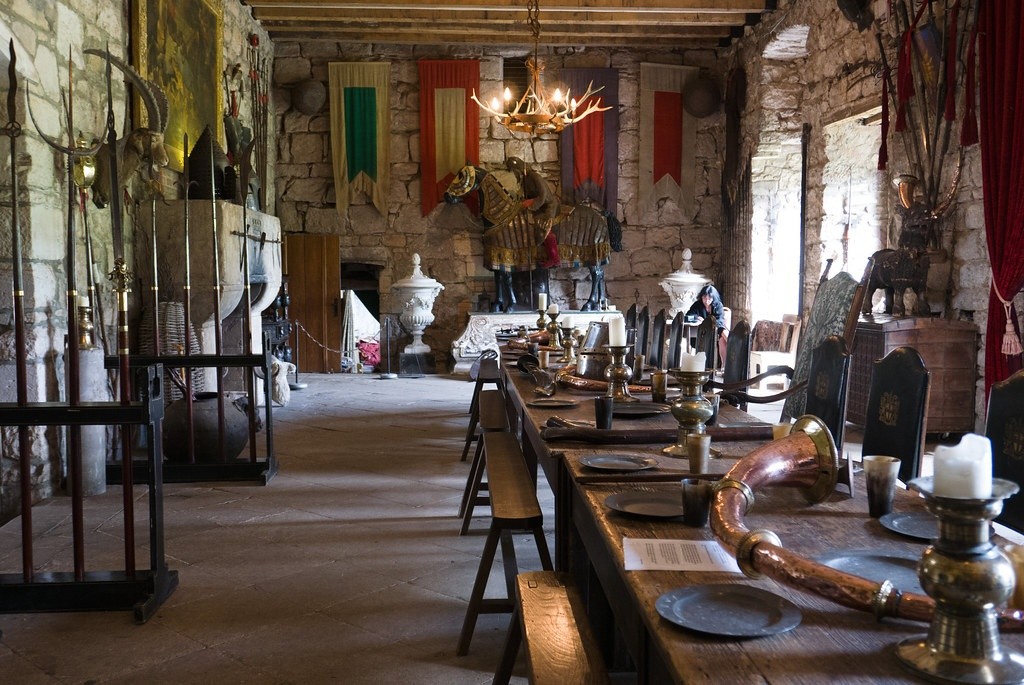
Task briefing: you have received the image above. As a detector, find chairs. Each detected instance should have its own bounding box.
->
[457,431,554,657]
[625,304,1024,534]
[492,571,610,685]
[469,360,503,413]
[458,390,508,520]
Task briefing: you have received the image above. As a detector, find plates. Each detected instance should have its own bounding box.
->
[879,511,996,540]
[578,454,658,472]
[605,490,684,522]
[654,582,802,637]
[526,398,579,407]
[809,548,928,596]
[612,403,672,419]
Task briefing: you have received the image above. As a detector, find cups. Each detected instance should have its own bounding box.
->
[634,354,645,379]
[687,434,711,474]
[538,350,549,368]
[650,373,668,403]
[594,395,613,430]
[681,479,712,528]
[772,423,793,441]
[863,455,901,518]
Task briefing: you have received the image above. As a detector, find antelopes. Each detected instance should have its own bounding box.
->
[82,48,170,210]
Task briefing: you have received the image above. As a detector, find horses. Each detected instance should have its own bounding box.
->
[444,163,623,313]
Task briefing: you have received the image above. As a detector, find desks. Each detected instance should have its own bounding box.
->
[494,329,1024,685]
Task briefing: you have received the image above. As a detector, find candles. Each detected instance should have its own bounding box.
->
[539,292,548,311]
[934,433,996,501]
[548,304,559,314]
[609,314,625,346]
[681,351,706,371]
[562,316,575,329]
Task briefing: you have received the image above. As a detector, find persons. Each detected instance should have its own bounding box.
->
[685,286,724,369]
[507,157,561,268]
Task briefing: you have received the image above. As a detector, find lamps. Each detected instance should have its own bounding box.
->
[471,0,613,135]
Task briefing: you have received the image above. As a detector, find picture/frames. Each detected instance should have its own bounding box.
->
[128,0,223,176]
